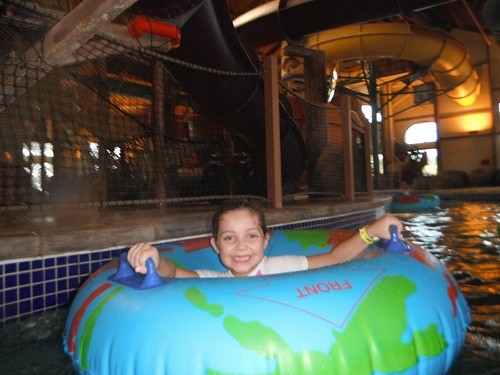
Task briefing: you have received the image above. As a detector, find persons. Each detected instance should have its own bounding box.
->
[127,200,403,277]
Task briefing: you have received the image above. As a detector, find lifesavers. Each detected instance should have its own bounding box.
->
[389,193,441,213]
[60,225,470,375]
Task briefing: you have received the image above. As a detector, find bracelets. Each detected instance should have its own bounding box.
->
[358,224,375,244]
